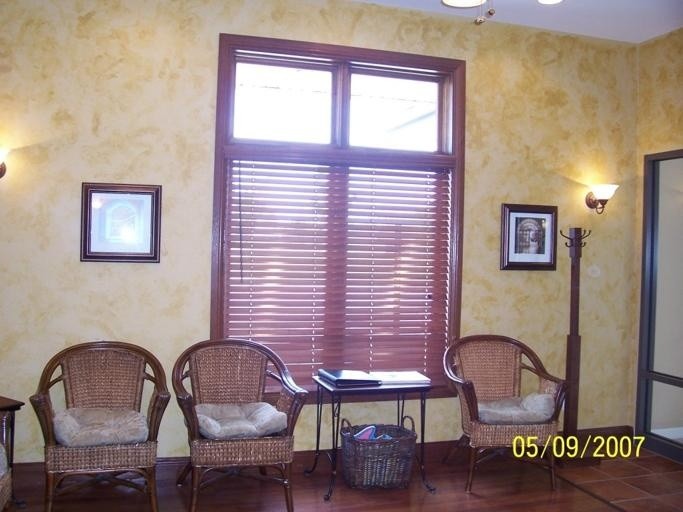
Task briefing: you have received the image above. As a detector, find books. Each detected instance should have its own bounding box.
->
[369,370,431,385]
[317,366,381,388]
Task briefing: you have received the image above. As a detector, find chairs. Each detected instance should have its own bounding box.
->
[172,337,307,511]
[441,333,570,493]
[27,339,171,511]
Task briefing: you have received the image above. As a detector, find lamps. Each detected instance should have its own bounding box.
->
[585,182,621,216]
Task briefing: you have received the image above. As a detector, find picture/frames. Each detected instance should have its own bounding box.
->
[500,202,558,273]
[78,181,162,265]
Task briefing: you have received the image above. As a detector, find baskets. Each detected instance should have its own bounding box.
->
[339,414,417,490]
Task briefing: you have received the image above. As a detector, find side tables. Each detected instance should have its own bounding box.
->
[303,375,436,499]
